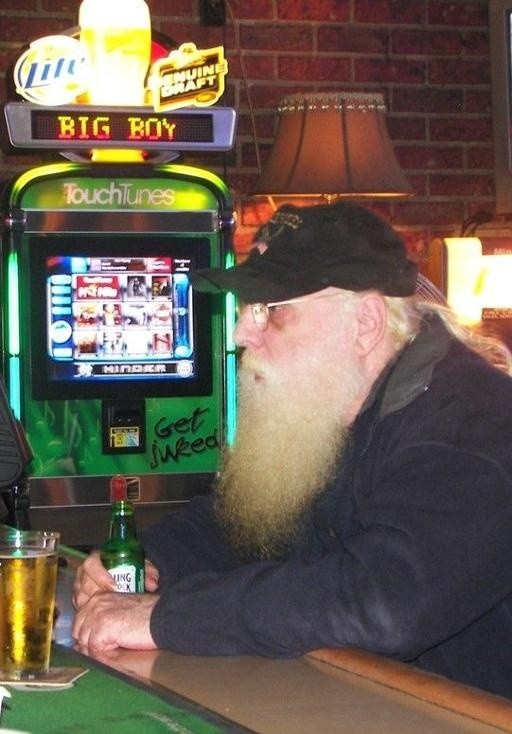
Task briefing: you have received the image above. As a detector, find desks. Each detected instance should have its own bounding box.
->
[0,544,512,733]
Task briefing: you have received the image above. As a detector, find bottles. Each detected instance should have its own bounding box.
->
[100,499,145,597]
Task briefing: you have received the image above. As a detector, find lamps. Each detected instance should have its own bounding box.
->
[241,92,420,207]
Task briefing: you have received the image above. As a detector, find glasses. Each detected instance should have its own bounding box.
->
[235,292,340,331]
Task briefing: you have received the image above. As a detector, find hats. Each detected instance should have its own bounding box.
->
[193,201,416,303]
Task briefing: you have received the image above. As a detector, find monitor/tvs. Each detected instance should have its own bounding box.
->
[35,239,200,388]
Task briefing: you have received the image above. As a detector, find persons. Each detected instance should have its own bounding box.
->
[68,199,511,703]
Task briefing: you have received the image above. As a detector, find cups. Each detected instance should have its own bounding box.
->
[0,529,61,682]
